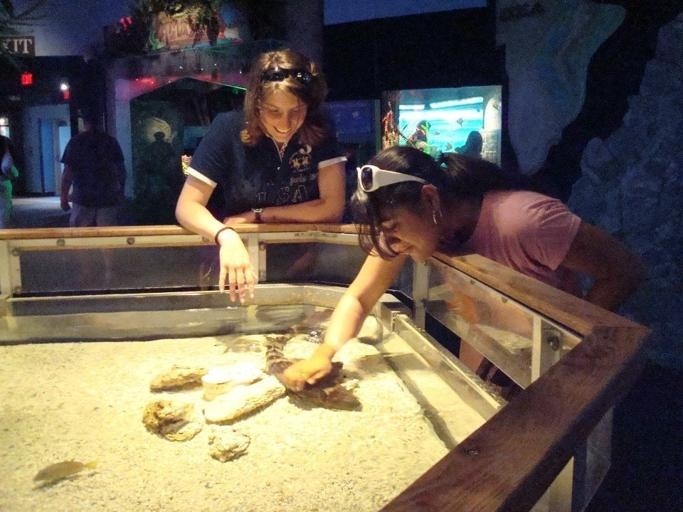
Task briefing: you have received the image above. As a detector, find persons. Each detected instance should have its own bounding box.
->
[279,144,645,405]
[172,49,349,308]
[455,131,483,160]
[406,120,431,152]
[0,134,17,228]
[57,110,125,225]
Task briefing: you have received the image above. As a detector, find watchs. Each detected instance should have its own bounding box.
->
[251,207,263,223]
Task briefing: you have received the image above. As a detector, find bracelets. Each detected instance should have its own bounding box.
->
[214,226,237,246]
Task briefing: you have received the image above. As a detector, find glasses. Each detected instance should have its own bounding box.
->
[257,66,313,83]
[355,166,428,193]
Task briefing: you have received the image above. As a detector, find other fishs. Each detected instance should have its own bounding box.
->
[201,360,343,426]
[32,458,95,485]
[264,334,361,412]
